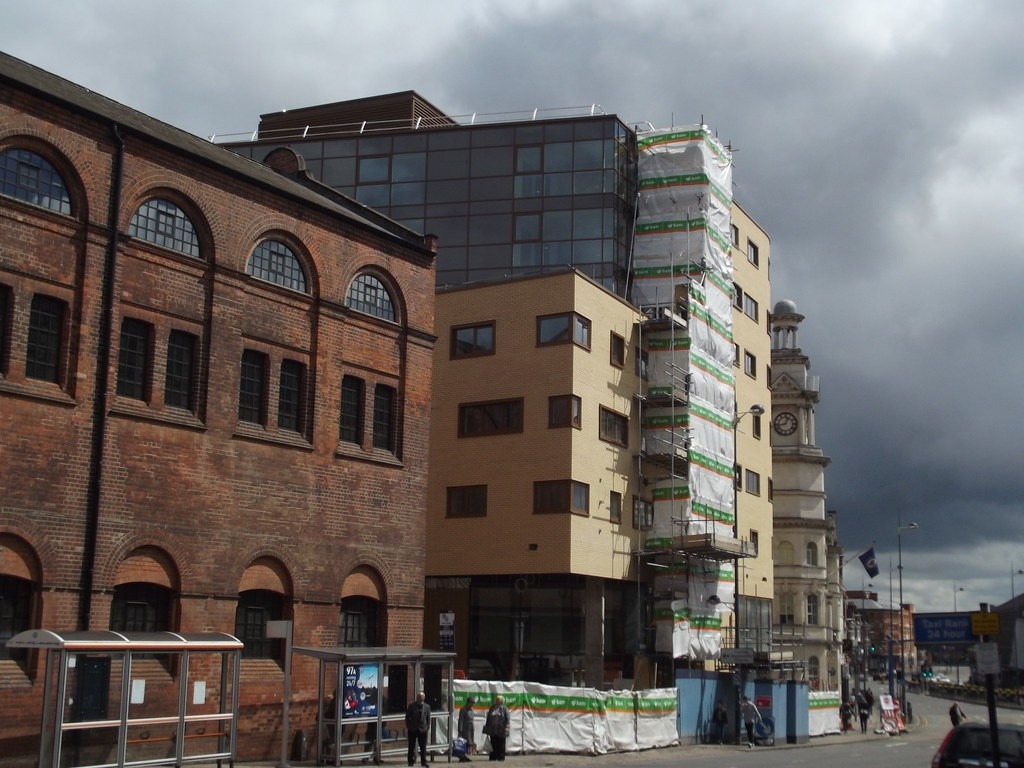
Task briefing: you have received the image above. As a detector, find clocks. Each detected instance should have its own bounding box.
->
[774,411,798,436]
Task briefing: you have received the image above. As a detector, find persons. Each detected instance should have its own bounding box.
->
[405,692,430,766]
[839,693,874,734]
[713,700,728,745]
[326,690,346,765]
[362,695,385,763]
[741,697,763,747]
[950,703,967,727]
[482,696,510,761]
[458,697,475,762]
[536,663,550,684]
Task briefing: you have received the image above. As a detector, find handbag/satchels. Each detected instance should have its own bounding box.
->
[451,737,468,756]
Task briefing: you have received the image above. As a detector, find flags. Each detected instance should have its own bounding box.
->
[859,547,879,578]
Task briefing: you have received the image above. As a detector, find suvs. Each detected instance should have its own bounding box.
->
[929,723,1024,768]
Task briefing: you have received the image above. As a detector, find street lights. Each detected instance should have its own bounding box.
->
[861,577,875,691]
[1011,561,1024,688]
[898,514,919,724]
[954,581,968,685]
